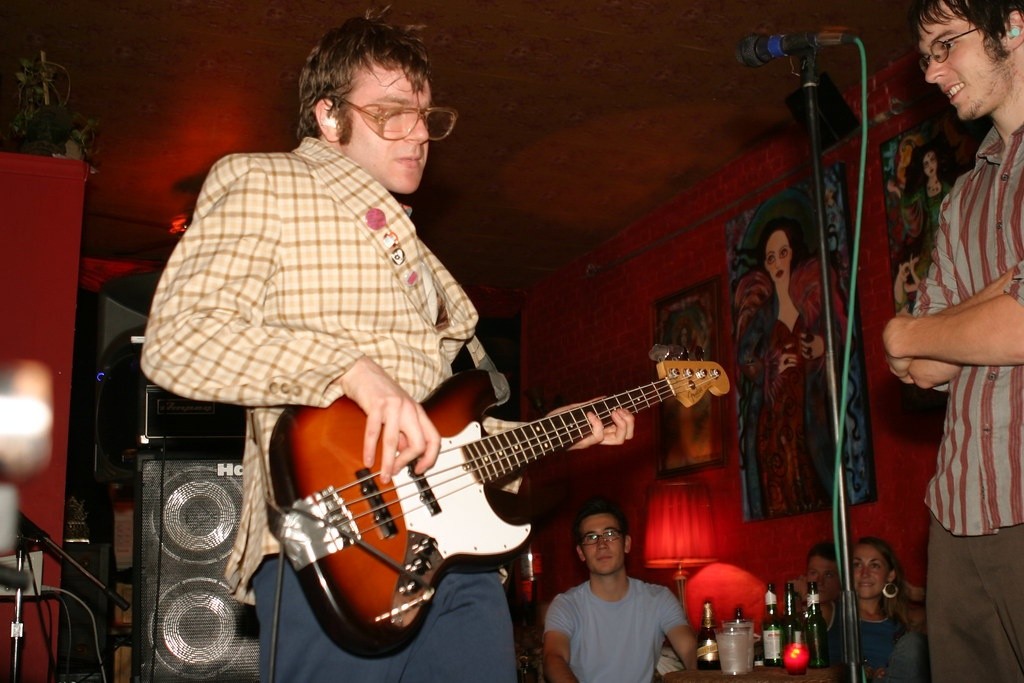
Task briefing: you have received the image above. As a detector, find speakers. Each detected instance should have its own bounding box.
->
[129,448,262,683]
[786,70,861,155]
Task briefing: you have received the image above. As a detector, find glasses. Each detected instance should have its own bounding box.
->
[919,22,990,74]
[579,528,625,546]
[334,95,458,141]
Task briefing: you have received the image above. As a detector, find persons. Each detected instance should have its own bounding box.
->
[882,1,1024,683]
[140,12,635,683]
[805,537,910,683]
[543,504,700,683]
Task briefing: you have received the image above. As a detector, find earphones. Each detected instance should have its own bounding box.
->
[321,117,339,128]
[1009,28,1019,39]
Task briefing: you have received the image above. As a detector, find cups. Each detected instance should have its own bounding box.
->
[715,628,749,675]
[722,622,754,672]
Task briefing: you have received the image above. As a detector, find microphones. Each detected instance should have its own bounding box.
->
[734,28,860,68]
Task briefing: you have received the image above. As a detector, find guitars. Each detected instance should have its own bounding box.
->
[267,340,732,662]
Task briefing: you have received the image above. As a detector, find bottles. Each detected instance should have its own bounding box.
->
[783,583,805,645]
[762,584,784,667]
[804,582,830,668]
[733,608,746,622]
[698,601,720,670]
